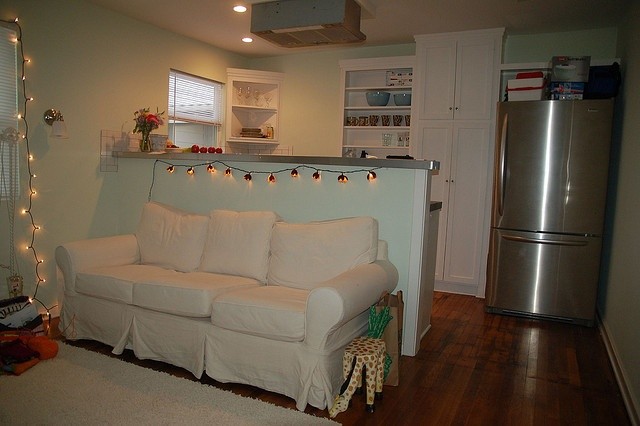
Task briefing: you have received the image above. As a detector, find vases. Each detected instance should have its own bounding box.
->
[141,131,151,152]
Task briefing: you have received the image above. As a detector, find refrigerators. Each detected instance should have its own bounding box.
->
[483,97,615,329]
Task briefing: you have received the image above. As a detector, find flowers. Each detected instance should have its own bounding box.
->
[132,108,165,134]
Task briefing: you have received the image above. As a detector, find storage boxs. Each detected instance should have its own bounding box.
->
[507,72,545,101]
[551,55,591,82]
[547,80,584,100]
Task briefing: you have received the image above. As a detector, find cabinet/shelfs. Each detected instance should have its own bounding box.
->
[408,122,498,300]
[337,55,414,159]
[226,66,281,144]
[415,27,504,121]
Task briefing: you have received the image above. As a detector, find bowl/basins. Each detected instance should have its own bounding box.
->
[393,93,411,106]
[165,148,183,153]
[366,91,391,106]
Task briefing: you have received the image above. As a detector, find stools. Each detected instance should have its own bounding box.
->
[330,337,388,414]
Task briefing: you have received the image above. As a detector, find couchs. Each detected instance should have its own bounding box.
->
[53,200,397,413]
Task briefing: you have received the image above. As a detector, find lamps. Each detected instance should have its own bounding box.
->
[42,106,70,140]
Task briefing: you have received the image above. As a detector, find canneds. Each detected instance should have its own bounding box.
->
[266,126,273,138]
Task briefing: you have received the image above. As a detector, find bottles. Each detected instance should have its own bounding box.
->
[345,148,356,158]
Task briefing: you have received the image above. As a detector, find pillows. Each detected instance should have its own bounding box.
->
[196,208,282,284]
[135,200,210,273]
[267,215,379,291]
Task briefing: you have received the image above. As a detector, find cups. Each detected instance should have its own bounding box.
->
[359,116,369,126]
[405,132,409,146]
[397,132,405,146]
[405,115,410,126]
[393,115,403,126]
[346,117,359,126]
[370,115,379,126]
[381,115,391,126]
[382,133,392,146]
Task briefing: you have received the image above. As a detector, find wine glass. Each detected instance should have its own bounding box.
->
[237,86,273,107]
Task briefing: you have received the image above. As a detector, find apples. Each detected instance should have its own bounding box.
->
[201,146,208,153]
[208,146,215,153]
[191,145,200,153]
[216,148,222,154]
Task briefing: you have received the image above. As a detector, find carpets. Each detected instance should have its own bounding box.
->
[0,338,343,426]
